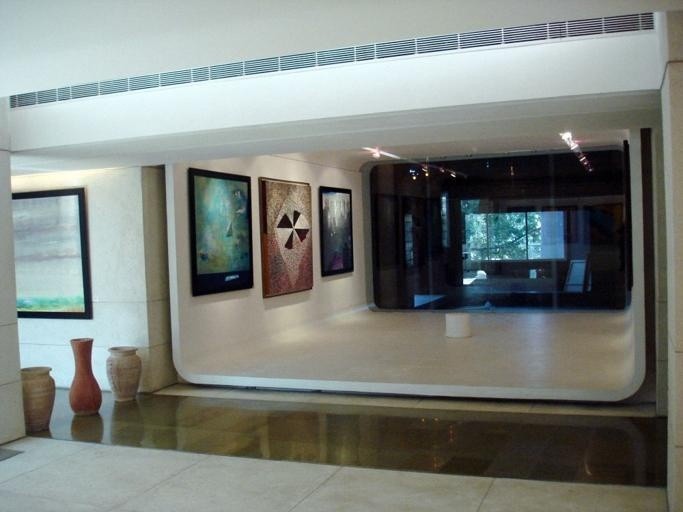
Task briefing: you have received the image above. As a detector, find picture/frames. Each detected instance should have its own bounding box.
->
[185,163,254,297]
[316,182,354,279]
[10,185,93,323]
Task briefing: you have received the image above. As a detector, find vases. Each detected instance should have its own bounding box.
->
[102,344,144,404]
[66,334,104,417]
[19,364,58,435]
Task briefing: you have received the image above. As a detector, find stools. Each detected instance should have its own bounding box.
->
[445,312,472,337]
